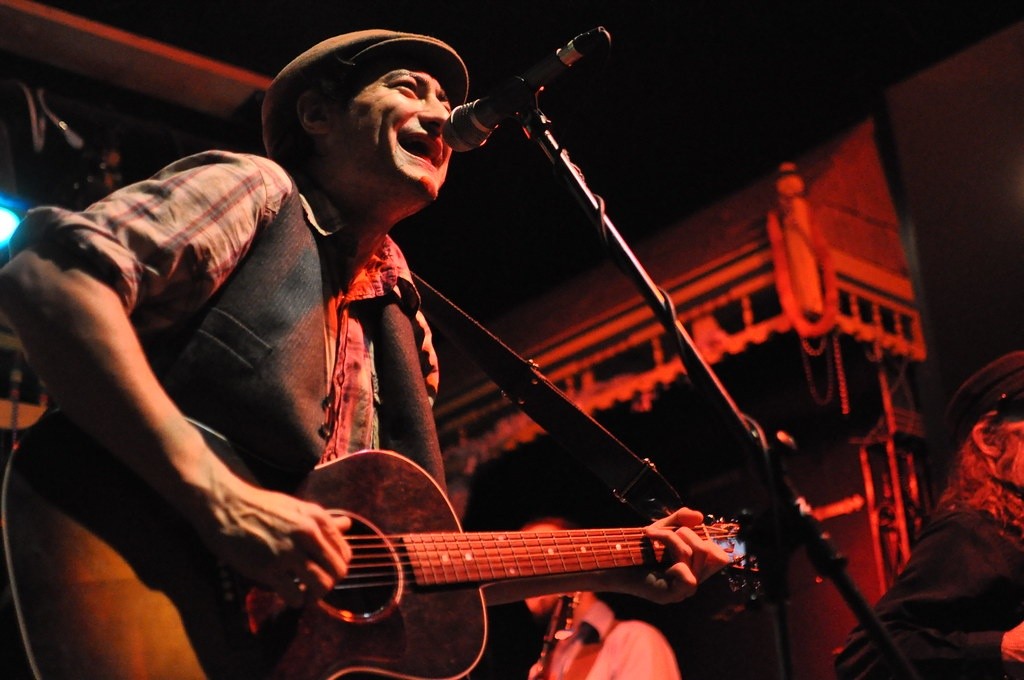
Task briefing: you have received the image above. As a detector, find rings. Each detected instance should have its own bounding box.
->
[288,579,299,585]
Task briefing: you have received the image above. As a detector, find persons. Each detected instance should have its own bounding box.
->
[0,29,729,680]
[833,348,1024,679]
[519,520,681,680]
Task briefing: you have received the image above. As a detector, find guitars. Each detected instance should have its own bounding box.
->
[0,396,763,680]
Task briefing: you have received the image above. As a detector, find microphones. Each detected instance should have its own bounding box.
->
[442,26,606,152]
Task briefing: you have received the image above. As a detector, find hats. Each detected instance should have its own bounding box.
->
[261,28,469,169]
[943,350,1024,445]
